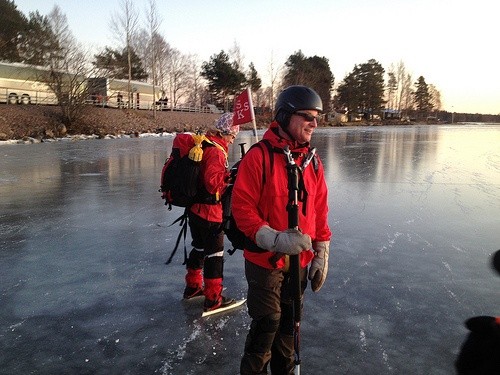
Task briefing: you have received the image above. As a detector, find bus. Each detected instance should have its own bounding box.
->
[87,76,167,110]
[0,62,90,106]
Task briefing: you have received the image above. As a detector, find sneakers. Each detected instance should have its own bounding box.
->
[183,286,204,299]
[204,296,236,311]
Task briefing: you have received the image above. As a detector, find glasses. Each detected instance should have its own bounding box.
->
[293,111,321,123]
[230,134,236,139]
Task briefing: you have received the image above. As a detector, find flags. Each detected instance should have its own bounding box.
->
[231,89,252,126]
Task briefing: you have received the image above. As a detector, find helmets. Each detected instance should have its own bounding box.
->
[275,85,323,113]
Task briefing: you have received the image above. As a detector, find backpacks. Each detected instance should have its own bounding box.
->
[162,140,220,208]
[222,138,319,249]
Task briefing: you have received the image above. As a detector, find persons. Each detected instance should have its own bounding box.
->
[116,92,123,107]
[229,86,332,375]
[159,96,168,107]
[454,249,500,375]
[183,113,239,312]
[91,90,110,105]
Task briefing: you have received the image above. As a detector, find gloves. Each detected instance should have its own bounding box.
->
[255,224,312,255]
[308,240,330,292]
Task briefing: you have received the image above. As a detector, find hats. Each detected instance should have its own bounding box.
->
[216,112,239,134]
